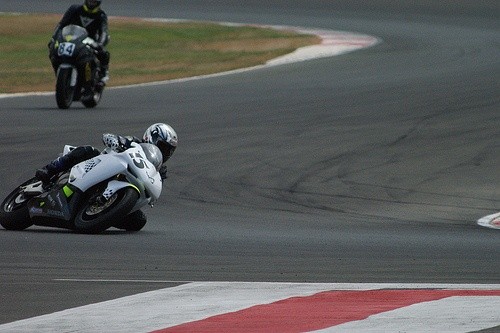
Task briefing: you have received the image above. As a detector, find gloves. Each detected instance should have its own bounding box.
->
[91,43,102,50]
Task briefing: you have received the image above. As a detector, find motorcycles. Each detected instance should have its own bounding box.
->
[47,24,104,110]
[0,138,168,234]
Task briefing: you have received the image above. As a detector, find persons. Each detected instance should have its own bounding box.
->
[36,120,178,231]
[47,0,110,95]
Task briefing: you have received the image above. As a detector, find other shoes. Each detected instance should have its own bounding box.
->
[97,73,108,85]
[35,166,50,182]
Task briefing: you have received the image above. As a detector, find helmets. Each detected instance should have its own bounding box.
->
[142,123,179,164]
